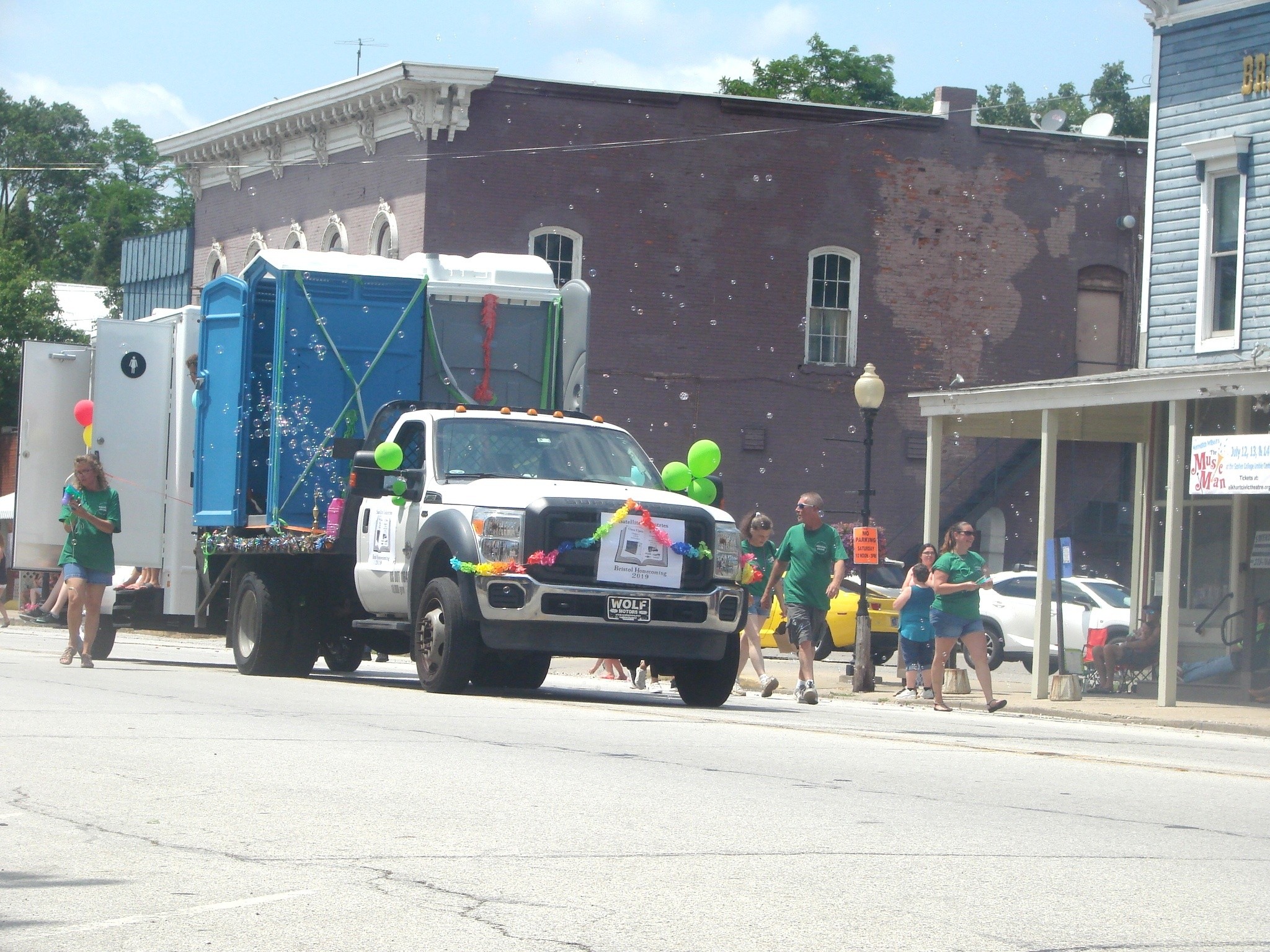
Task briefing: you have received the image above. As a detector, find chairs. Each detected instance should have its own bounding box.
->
[477,453,513,475]
[1063,620,1152,697]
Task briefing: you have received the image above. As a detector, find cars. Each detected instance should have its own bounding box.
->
[738,571,898,664]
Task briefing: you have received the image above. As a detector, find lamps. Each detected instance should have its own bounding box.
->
[907,433,928,459]
[1114,216,1137,231]
[948,375,964,389]
[740,426,765,451]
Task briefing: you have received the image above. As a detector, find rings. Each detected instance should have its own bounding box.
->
[78,503,81,507]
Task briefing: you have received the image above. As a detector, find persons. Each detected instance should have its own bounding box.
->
[113,567,161,591]
[929,522,1007,712]
[892,564,934,701]
[1086,602,1162,693]
[57,454,121,668]
[731,512,788,697]
[897,544,938,686]
[18,569,68,623]
[185,351,198,412]
[0,531,10,628]
[760,491,849,704]
[1177,601,1270,683]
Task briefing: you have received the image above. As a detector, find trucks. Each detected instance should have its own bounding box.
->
[11,250,750,707]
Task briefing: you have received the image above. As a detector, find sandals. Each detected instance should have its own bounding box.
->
[933,701,953,712]
[59,646,77,664]
[81,653,94,668]
[987,699,1007,713]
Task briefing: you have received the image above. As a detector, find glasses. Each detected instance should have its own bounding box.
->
[1145,610,1159,615]
[75,467,96,477]
[922,551,937,555]
[187,372,197,377]
[958,531,976,536]
[796,503,818,509]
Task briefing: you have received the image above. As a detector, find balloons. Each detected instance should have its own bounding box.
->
[661,439,721,505]
[75,400,93,447]
[373,441,404,470]
[392,479,406,507]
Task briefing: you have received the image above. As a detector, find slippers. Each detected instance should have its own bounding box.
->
[113,583,161,590]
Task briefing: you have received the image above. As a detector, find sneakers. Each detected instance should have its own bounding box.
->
[649,682,663,693]
[892,686,935,700]
[634,667,647,690]
[793,681,818,705]
[761,676,779,697]
[20,606,61,624]
[729,680,746,696]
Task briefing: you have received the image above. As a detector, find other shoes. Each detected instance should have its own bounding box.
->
[599,674,627,680]
[1087,685,1114,694]
[362,652,371,661]
[376,654,389,662]
[1177,661,1184,683]
[1,622,10,628]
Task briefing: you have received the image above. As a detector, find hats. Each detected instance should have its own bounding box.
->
[1143,601,1161,611]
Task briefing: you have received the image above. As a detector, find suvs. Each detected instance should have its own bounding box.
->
[959,563,1132,675]
[842,559,906,598]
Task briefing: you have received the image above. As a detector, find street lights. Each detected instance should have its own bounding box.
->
[846,362,884,676]
